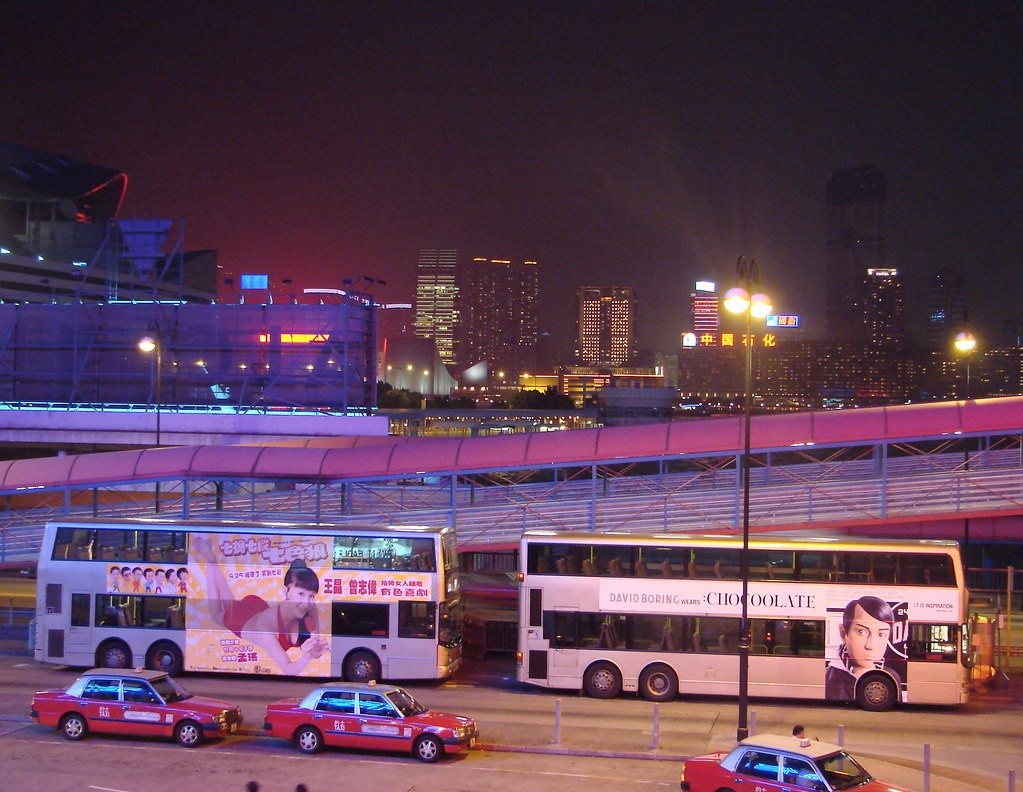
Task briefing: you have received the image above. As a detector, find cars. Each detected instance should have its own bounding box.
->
[27,667,243,747]
[680,734,903,792]
[262,679,478,764]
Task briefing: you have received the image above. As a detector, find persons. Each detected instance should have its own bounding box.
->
[792,725,804,739]
[796,763,815,787]
[192,537,328,676]
[109,565,189,593]
[826,596,903,702]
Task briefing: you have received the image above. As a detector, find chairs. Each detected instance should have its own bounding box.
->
[540,555,721,580]
[601,620,728,651]
[51,544,185,629]
[392,552,437,572]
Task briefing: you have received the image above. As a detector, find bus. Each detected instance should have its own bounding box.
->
[34,522,462,683]
[518,529,973,711]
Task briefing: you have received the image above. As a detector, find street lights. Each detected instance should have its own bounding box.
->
[954,322,977,470]
[138,320,163,515]
[723,288,772,743]
